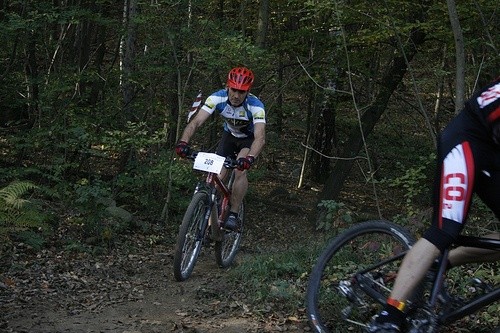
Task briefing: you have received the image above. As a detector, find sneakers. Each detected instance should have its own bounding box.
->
[224,212,238,229]
[204,224,214,244]
[364,315,406,333]
[422,261,450,303]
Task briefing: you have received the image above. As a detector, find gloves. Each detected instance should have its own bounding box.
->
[238,155,255,171]
[176,140,188,158]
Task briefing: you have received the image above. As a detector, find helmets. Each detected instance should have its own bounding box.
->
[227,67,254,91]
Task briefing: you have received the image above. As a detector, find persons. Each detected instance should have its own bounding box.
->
[175,66,266,245]
[373,81,500,333]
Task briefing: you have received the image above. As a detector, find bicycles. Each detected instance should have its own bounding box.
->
[173,145,254,282]
[306,219,500,333]
